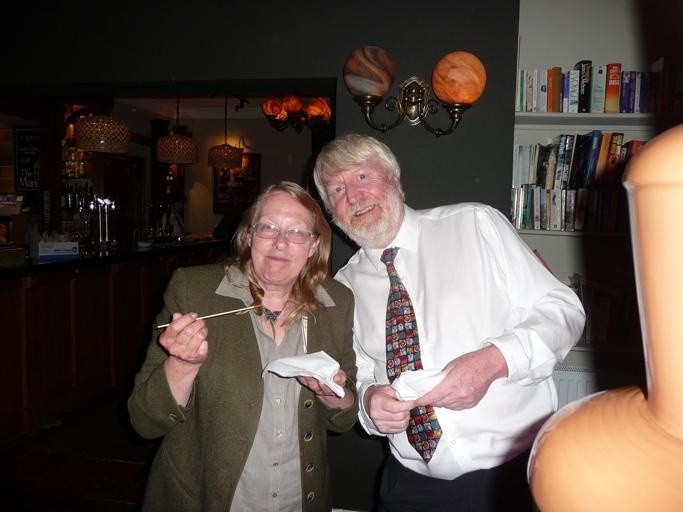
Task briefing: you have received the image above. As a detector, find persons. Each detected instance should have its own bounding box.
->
[157,195,185,232]
[217,185,249,258]
[313,135,587,511]
[128,181,358,512]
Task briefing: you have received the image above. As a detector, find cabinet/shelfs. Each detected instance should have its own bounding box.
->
[512,109,656,238]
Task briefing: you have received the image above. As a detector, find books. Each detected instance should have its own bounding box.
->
[510,53,683,348]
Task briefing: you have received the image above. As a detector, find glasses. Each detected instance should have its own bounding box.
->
[253,222,318,244]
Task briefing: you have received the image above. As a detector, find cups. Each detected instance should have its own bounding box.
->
[135,224,156,247]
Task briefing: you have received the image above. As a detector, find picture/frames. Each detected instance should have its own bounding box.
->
[12,126,41,192]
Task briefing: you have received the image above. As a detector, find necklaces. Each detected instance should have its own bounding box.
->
[264,308,282,341]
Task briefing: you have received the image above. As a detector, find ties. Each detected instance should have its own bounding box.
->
[380,247,443,464]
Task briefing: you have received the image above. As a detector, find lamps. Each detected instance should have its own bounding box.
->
[260,92,332,135]
[154,98,200,165]
[206,95,243,170]
[342,44,488,138]
[73,115,130,154]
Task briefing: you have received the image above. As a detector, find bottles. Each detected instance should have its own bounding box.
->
[60,181,90,209]
